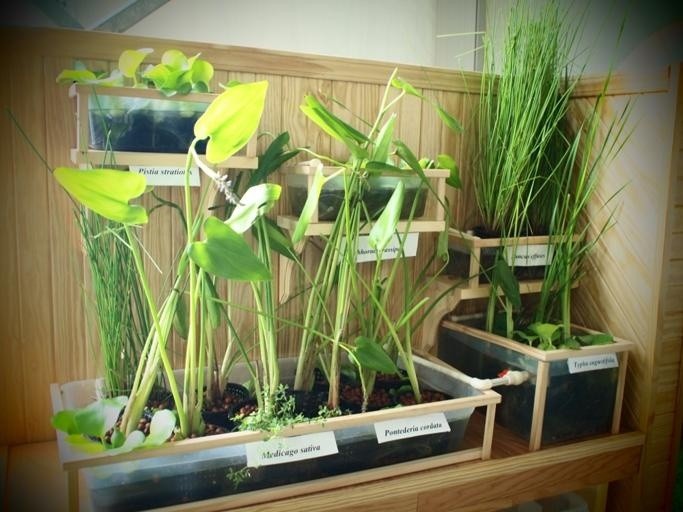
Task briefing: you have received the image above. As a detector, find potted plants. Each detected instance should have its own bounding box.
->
[436,81,620,448]
[437,0,643,283]
